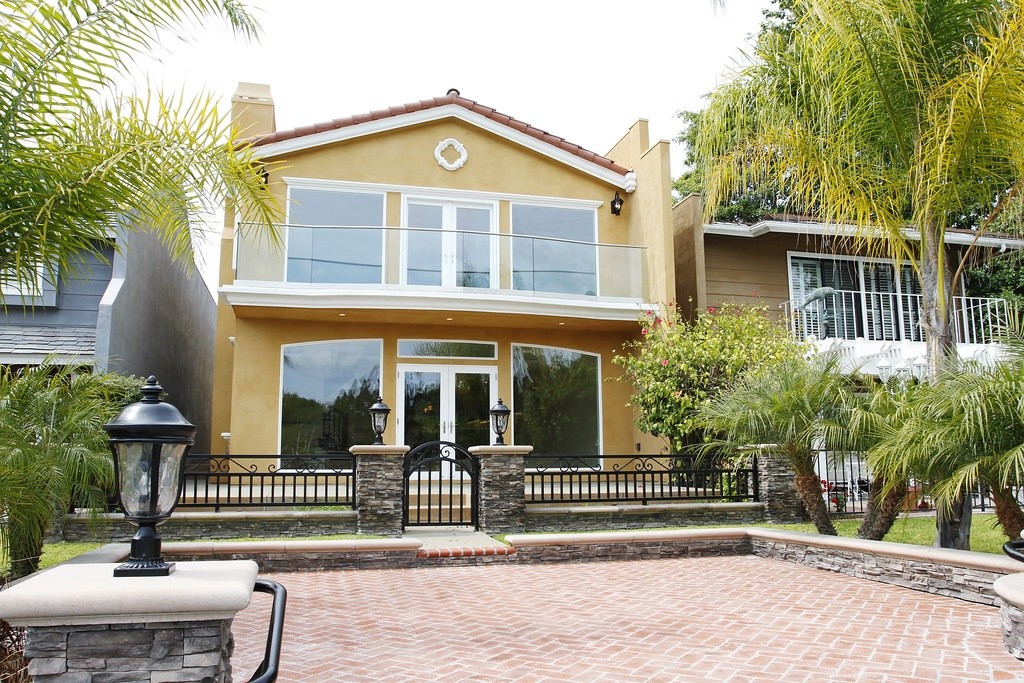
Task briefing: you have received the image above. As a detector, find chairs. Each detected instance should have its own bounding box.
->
[845,462,871,502]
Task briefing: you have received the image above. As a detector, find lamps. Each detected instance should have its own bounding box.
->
[103,375,197,577]
[488,398,511,446]
[256,167,269,190]
[611,191,624,216]
[368,396,391,445]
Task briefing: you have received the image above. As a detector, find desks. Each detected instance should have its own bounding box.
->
[993,572,1024,659]
